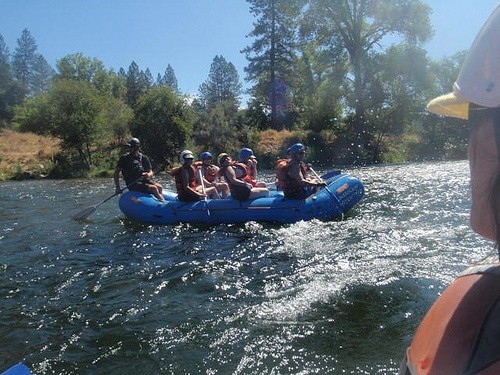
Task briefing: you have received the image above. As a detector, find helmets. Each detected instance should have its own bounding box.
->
[180,149,194,164]
[239,148,256,162]
[126,137,140,148]
[286,142,305,153]
[217,152,229,164]
[200,152,212,162]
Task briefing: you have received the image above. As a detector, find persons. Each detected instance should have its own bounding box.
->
[114,138,165,201]
[400,6,500,375]
[172,148,269,201]
[276,143,326,198]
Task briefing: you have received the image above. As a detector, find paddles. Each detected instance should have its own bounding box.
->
[198,169,211,227]
[309,167,346,212]
[74,176,144,221]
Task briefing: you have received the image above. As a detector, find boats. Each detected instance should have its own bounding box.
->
[119,169,364,223]
